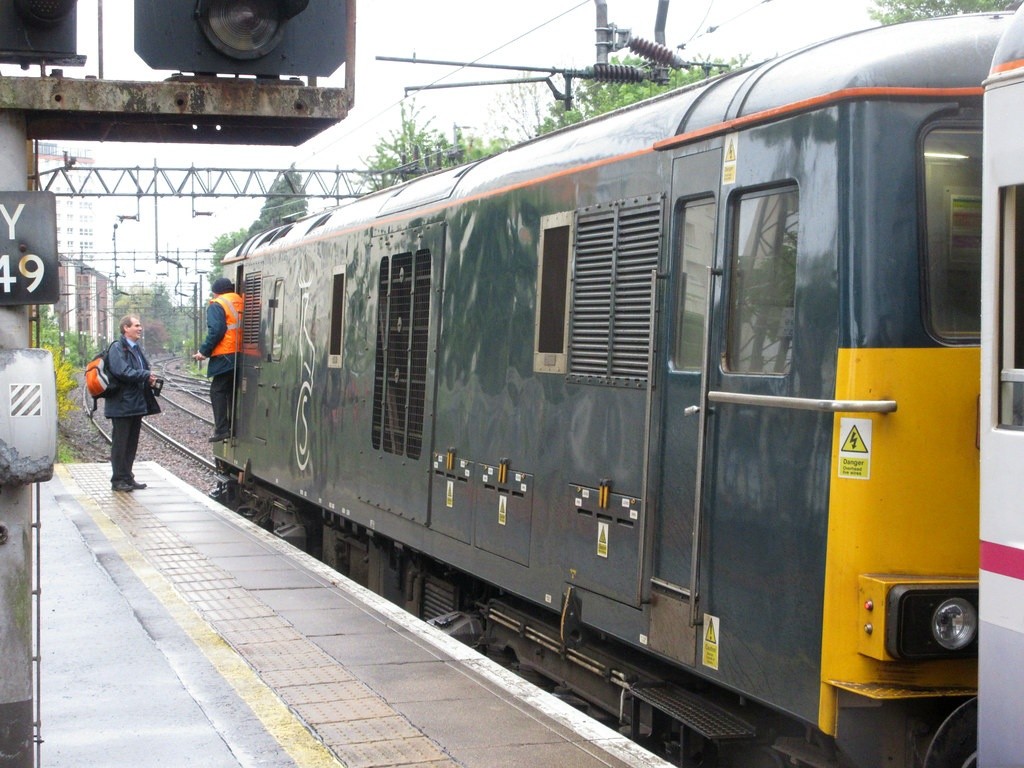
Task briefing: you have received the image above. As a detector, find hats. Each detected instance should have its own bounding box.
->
[211,277,234,295]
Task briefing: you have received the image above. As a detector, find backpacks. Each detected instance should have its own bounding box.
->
[85,341,128,399]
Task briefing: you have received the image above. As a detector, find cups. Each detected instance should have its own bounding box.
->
[154,379,164,396]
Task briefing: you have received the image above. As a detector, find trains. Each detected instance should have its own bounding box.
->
[211,0,1024,768]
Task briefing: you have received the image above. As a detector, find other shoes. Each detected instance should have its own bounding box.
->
[126,479,152,489]
[209,429,231,442]
[112,481,133,492]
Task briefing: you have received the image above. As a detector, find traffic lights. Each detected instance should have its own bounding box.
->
[0,0,88,67]
[134,0,352,78]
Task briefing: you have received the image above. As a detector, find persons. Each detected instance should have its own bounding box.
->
[192,277,243,443]
[104,315,161,491]
